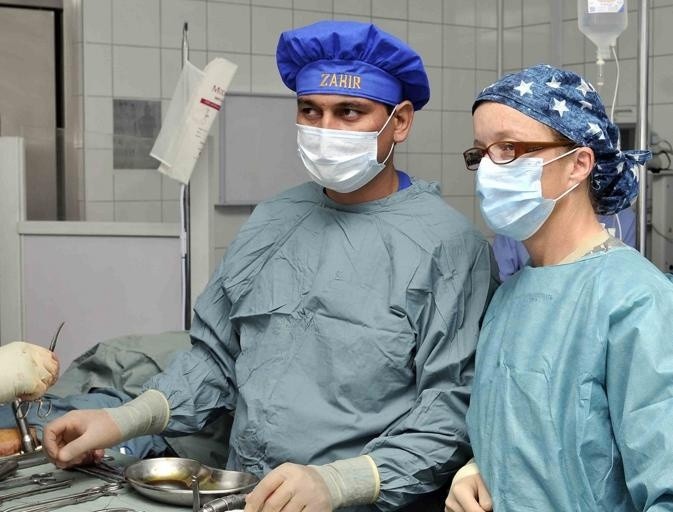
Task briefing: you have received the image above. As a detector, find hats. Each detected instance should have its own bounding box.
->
[274,18,431,117]
[467,60,651,219]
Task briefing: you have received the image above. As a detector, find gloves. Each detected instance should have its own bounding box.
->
[41,388,171,472]
[241,450,382,512]
[442,459,493,512]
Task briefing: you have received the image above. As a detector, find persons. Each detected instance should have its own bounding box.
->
[440,64,672,512]
[41,22,490,512]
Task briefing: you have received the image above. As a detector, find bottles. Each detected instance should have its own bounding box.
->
[574,0,629,61]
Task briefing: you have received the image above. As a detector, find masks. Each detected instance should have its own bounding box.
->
[293,104,404,193]
[472,144,589,244]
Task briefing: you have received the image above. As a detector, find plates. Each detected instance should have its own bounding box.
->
[122,455,261,507]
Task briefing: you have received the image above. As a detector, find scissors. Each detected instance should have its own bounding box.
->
[15,320,66,419]
[0,471,125,508]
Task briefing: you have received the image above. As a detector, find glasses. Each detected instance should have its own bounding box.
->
[462,138,571,173]
